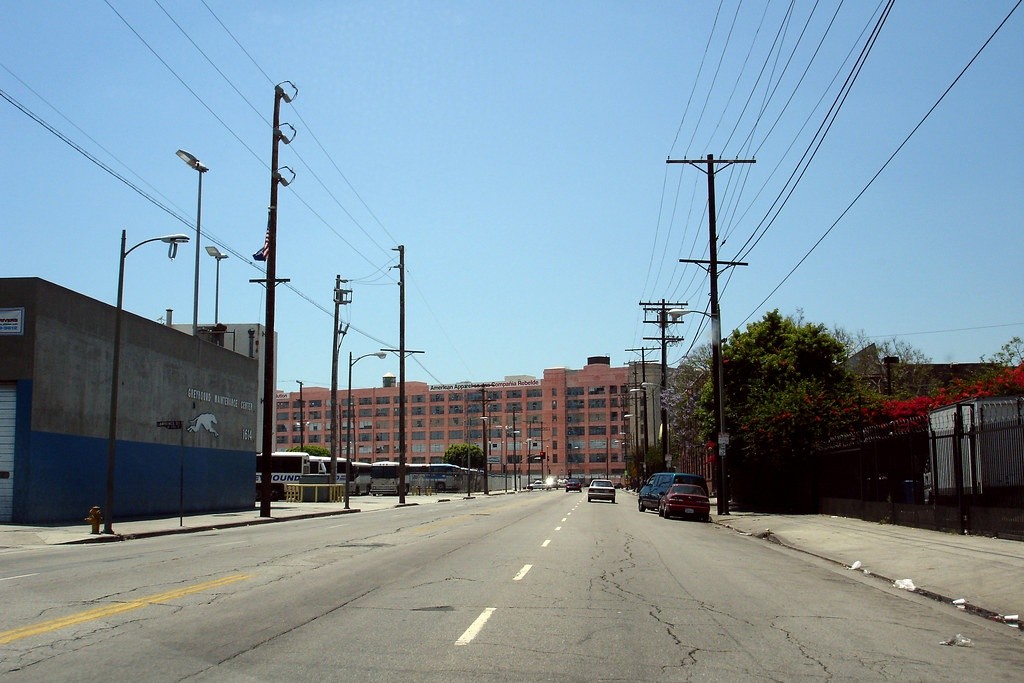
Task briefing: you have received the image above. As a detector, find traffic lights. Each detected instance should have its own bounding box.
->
[540,451,546,460]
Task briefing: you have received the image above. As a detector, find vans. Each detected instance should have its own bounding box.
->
[637,472,710,513]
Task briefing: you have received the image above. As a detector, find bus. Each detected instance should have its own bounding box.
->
[310,456,357,492]
[352,460,484,495]
[255,451,311,502]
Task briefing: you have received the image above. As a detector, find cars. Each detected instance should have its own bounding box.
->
[587,479,617,503]
[657,483,710,522]
[526,475,583,493]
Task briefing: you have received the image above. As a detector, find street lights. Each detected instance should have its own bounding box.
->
[343,352,387,510]
[668,308,730,517]
[467,416,488,497]
[504,430,521,493]
[205,245,230,323]
[102,228,190,535]
[175,149,210,337]
[883,354,901,399]
[641,382,671,473]
[630,387,658,471]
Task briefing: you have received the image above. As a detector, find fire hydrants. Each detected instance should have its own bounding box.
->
[84,506,105,533]
